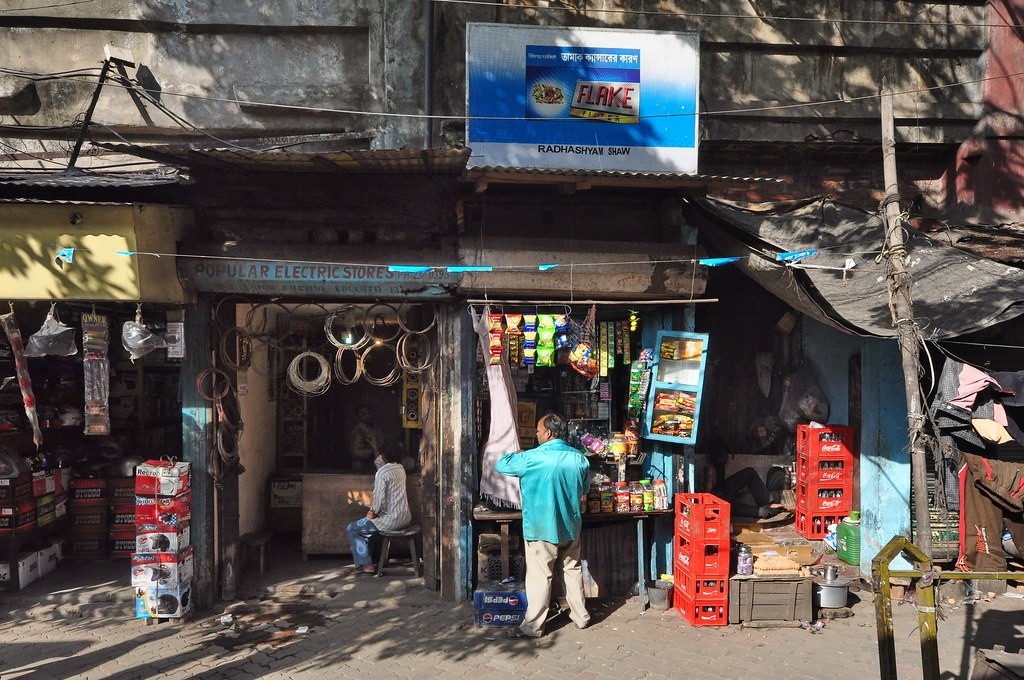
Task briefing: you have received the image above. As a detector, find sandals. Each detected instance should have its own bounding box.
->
[502,628,534,639]
[565,609,587,628]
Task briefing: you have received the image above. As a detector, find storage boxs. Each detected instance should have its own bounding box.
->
[107,506,135,533]
[795,507,850,540]
[135,490,192,525]
[136,579,191,619]
[518,402,549,426]
[674,585,728,626]
[797,452,853,485]
[131,547,193,586]
[136,459,193,497]
[473,582,528,627]
[796,480,852,512]
[110,374,182,449]
[796,424,853,456]
[108,479,136,505]
[107,532,136,558]
[674,566,729,600]
[518,427,540,451]
[136,520,190,553]
[674,493,731,539]
[729,579,812,625]
[0,467,107,591]
[675,532,730,575]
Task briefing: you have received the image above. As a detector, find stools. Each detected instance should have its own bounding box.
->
[376,520,421,578]
[239,533,272,580]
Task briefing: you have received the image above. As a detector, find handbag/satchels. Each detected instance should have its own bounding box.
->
[559,559,599,597]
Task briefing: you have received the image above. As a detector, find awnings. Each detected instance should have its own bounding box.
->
[88,141,473,185]
[701,193,1024,340]
[0,172,183,195]
[466,158,789,195]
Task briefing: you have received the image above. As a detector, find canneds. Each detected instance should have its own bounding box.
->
[0,481,35,527]
[72,487,136,551]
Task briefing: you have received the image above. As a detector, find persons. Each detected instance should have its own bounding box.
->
[346,403,412,578]
[695,465,769,520]
[493,415,593,642]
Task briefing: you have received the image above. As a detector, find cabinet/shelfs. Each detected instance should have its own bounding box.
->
[640,330,709,444]
[559,365,612,434]
[277,314,422,471]
[0,367,180,593]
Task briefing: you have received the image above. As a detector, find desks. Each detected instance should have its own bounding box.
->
[473,502,673,612]
[302,473,422,563]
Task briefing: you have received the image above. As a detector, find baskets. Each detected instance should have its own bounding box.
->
[487,551,525,581]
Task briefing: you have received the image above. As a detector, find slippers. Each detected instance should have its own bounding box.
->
[345,566,375,576]
